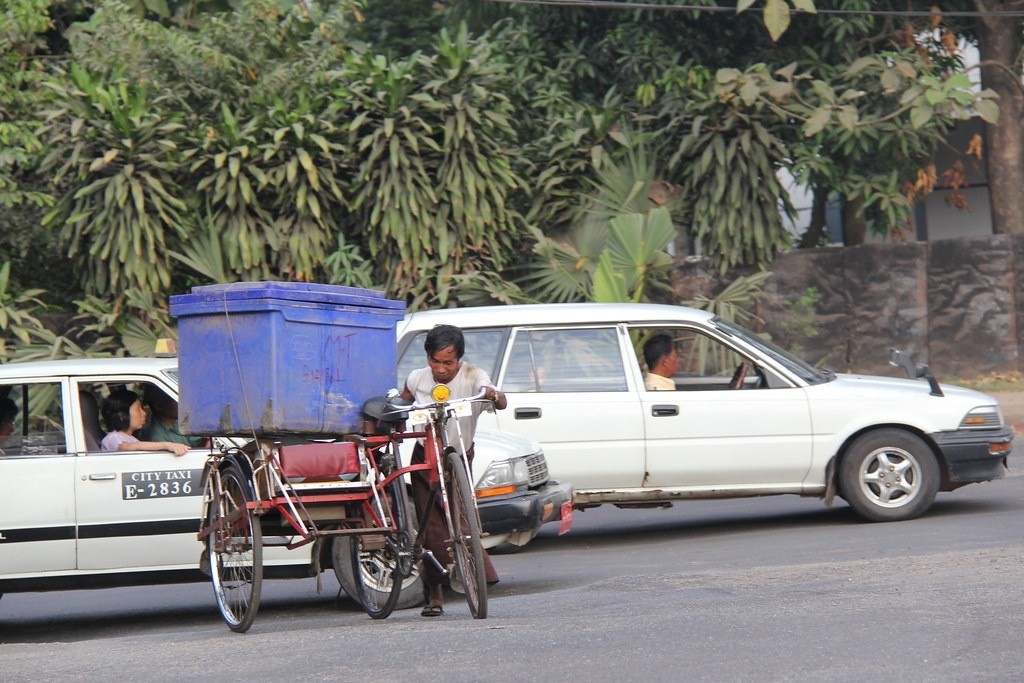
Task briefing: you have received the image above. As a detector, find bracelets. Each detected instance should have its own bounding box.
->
[492,390,498,402]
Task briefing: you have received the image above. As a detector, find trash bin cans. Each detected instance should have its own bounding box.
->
[168,279,407,436]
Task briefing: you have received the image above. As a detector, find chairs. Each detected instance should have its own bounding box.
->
[78,389,108,452]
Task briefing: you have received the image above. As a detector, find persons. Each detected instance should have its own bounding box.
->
[100,383,210,456]
[399,323,508,617]
[0,397,20,457]
[642,333,680,391]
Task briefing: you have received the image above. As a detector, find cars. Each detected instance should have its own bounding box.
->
[395,304,1015,522]
[0,339,575,611]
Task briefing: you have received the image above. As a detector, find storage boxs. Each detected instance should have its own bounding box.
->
[168,280,407,437]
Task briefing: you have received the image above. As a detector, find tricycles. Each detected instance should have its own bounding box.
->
[197,382,496,632]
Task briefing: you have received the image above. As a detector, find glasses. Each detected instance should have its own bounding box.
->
[0,420,15,426]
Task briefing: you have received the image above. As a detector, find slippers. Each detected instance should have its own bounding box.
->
[420,603,443,617]
